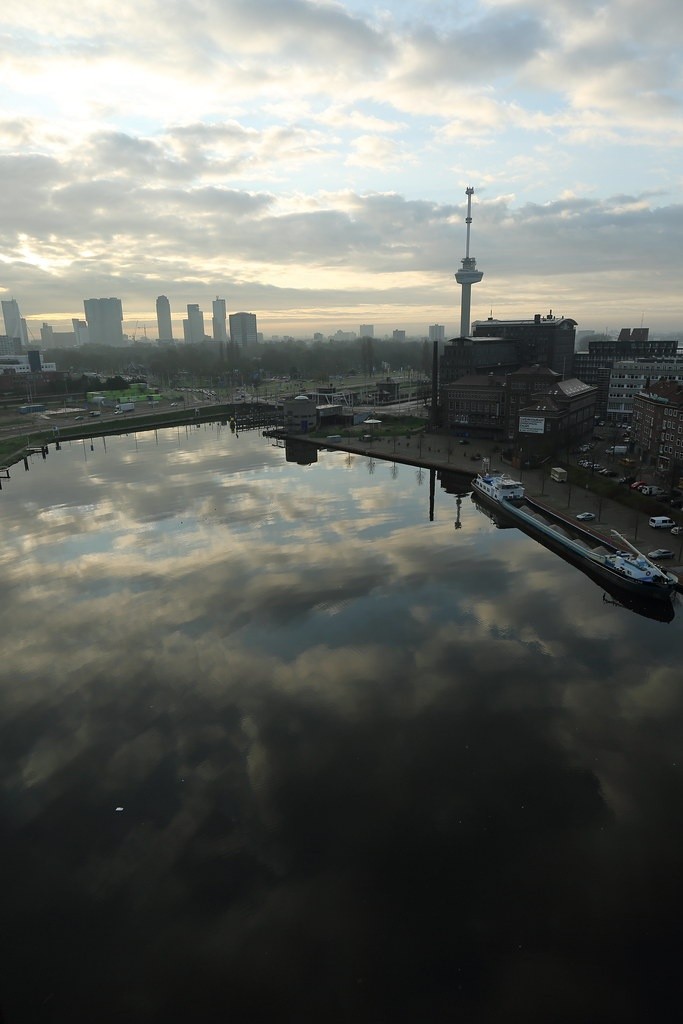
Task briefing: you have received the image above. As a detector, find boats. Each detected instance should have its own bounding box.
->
[469,456,679,600]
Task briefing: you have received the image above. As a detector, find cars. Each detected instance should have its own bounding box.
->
[576,458,683,508]
[174,387,217,397]
[171,402,178,407]
[617,422,632,444]
[572,441,596,454]
[576,512,596,522]
[647,548,676,561]
[670,526,683,535]
[74,416,84,420]
[598,420,605,426]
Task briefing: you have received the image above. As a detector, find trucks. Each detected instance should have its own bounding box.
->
[88,410,100,417]
[115,403,135,414]
[605,445,628,457]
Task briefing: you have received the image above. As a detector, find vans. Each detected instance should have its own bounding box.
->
[648,516,676,529]
